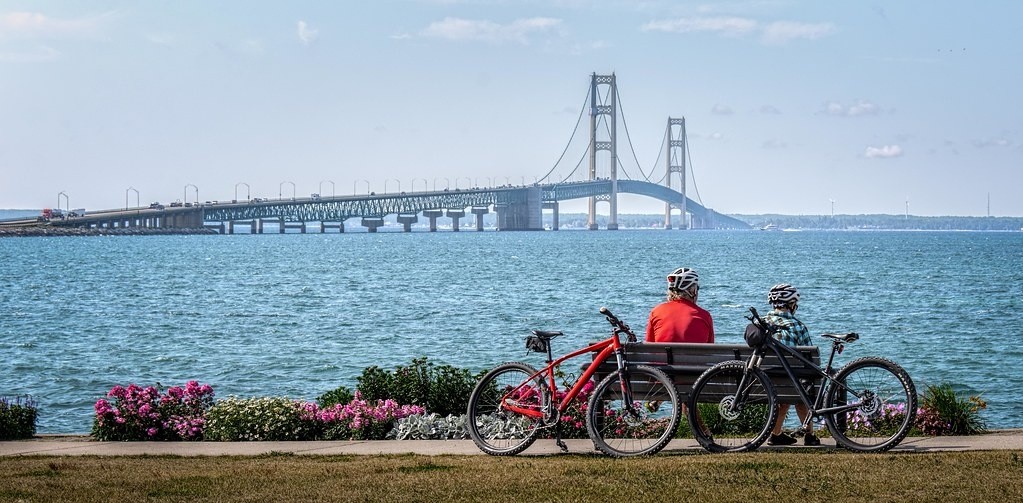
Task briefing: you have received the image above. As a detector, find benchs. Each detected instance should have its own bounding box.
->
[587,343,821,450]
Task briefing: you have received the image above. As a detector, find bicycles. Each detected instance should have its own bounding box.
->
[465,305,683,459]
[687,305,918,453]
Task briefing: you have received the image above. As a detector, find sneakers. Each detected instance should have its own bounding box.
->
[804,432,820,446]
[766,431,797,446]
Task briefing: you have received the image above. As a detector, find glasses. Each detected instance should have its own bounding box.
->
[697,285,699,291]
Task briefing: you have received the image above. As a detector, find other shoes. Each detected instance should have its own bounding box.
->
[646,399,661,414]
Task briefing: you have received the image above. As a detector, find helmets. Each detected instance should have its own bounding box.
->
[768,283,800,308]
[666,267,698,292]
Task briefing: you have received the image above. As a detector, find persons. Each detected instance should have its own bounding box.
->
[754,284,821,446]
[644,268,715,442]
